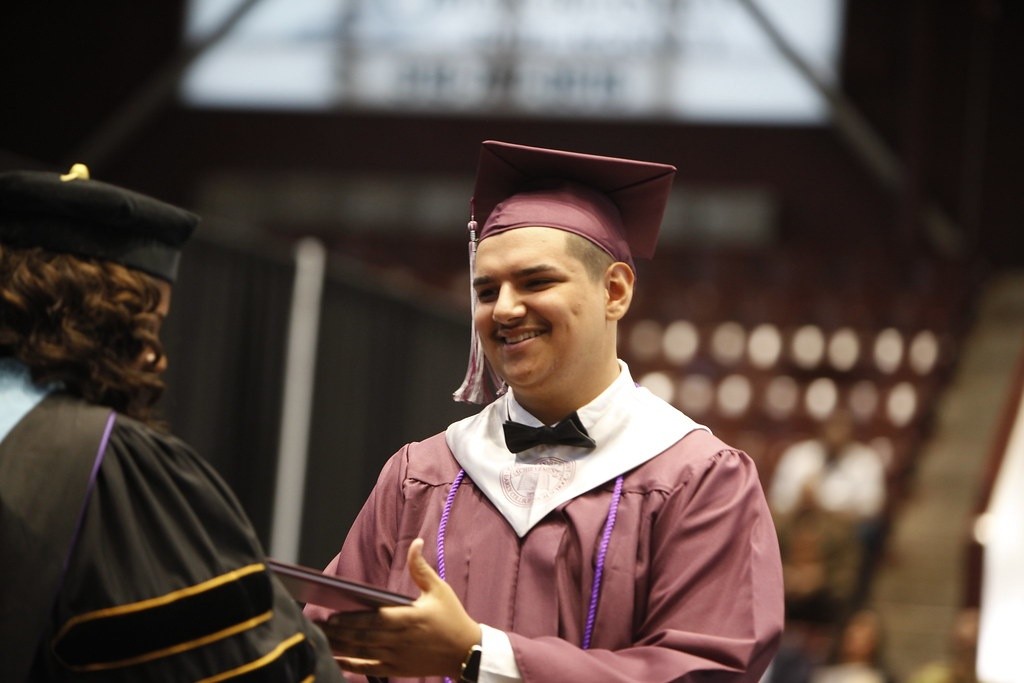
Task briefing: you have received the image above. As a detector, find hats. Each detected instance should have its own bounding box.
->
[0,163,200,286]
[452,141,676,404]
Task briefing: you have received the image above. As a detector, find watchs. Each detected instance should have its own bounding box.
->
[458,645,481,683]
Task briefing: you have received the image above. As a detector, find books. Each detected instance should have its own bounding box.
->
[267,559,417,611]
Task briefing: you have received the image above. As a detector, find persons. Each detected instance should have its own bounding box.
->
[0,161,348,683]
[300,140,786,683]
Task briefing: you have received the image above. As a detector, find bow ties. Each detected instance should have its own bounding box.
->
[501,409,597,454]
